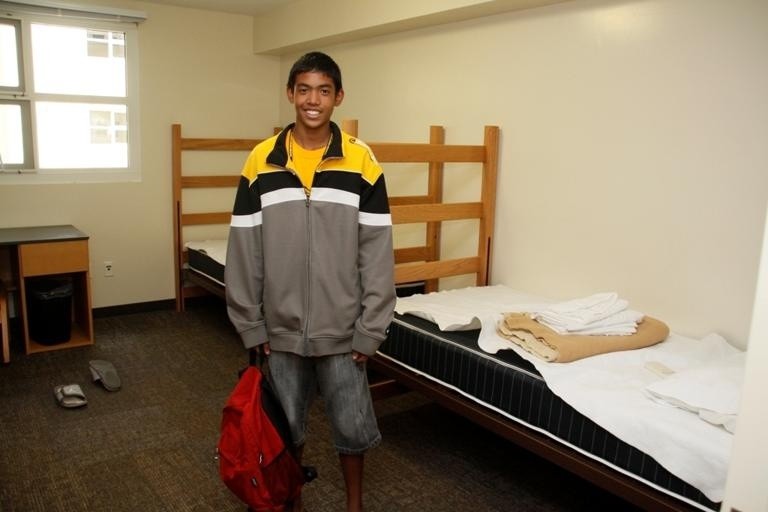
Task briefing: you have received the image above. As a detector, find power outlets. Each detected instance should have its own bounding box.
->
[104,261,112,276]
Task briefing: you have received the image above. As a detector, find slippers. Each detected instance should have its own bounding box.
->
[54,383,88,408]
[88,359,121,392]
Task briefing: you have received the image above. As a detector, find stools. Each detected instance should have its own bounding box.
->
[0,280,18,364]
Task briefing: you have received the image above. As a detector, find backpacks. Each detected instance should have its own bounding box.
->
[219,344,318,512]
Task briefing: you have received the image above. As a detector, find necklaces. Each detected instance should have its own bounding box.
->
[288,126,332,195]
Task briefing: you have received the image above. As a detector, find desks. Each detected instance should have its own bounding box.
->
[0,224,95,355]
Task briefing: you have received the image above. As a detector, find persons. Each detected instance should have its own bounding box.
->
[224,52,397,512]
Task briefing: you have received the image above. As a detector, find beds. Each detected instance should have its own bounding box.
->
[171,124,444,314]
[342,119,750,512]
